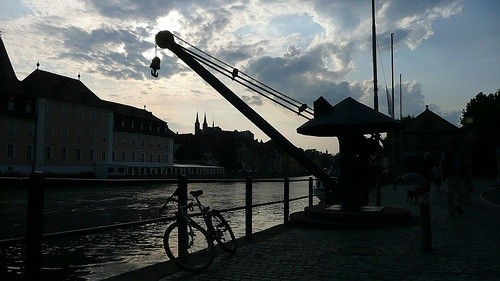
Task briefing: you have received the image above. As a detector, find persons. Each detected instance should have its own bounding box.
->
[420,148,473,216]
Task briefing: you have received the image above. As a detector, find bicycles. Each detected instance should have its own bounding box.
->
[158,183,238,273]
